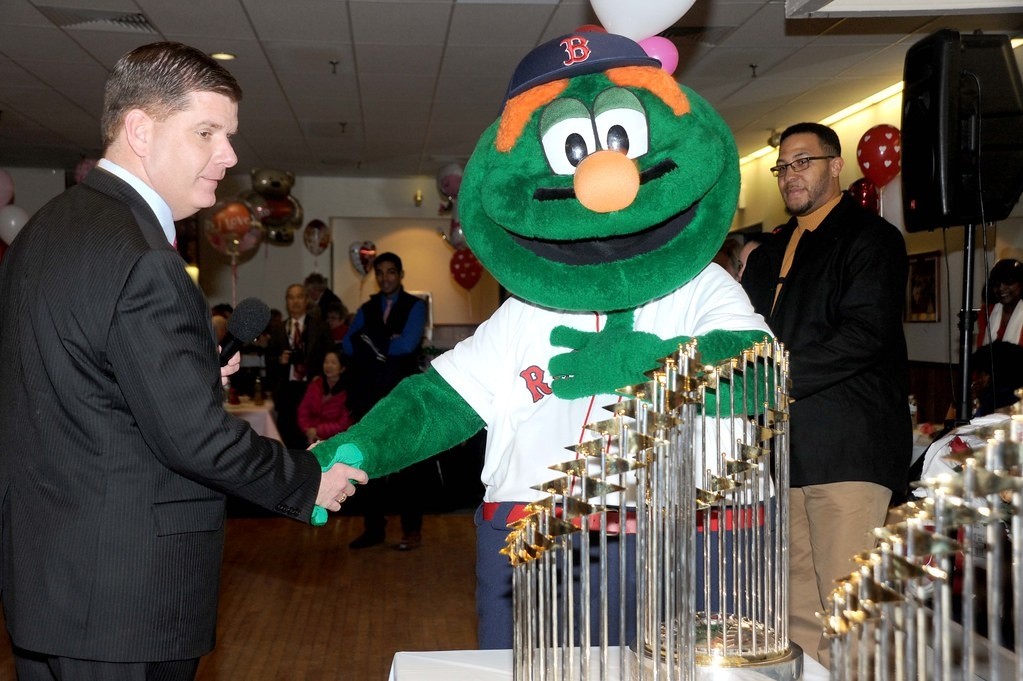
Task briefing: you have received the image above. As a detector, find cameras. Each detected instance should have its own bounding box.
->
[288,349,305,364]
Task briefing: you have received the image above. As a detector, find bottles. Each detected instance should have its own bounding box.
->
[908,395,918,429]
[254,376,263,405]
[228,389,236,405]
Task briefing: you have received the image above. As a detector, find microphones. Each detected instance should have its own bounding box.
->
[218,297,271,367]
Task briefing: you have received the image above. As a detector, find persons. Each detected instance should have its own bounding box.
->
[0,41,367,681]
[724,232,776,284]
[918,258,1023,499]
[349,252,428,551]
[740,122,914,681]
[212,273,354,447]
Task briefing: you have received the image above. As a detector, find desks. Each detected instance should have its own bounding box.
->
[910,425,944,466]
[388,646,830,681]
[222,396,285,447]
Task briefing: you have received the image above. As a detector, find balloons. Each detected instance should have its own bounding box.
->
[574,25,606,33]
[226,299,272,347]
[857,124,901,198]
[0,171,33,246]
[436,161,483,290]
[350,240,378,275]
[637,36,678,77]
[73,158,98,185]
[203,168,333,261]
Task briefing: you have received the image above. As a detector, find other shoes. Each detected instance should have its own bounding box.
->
[399,531,421,550]
[349,528,385,549]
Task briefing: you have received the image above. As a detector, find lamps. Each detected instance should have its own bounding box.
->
[414,189,424,208]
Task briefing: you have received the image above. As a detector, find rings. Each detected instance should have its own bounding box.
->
[338,493,347,503]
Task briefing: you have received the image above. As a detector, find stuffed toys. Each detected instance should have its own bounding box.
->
[308,30,789,649]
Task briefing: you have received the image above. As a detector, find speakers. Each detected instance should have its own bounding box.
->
[901,29,1023,233]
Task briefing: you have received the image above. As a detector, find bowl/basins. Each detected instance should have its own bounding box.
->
[239,396,249,403]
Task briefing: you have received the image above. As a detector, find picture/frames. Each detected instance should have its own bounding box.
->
[904,250,942,324]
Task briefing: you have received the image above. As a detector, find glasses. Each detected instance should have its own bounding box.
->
[770,155,836,178]
[988,276,1016,288]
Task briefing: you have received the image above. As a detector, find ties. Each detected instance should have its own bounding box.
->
[293,322,307,382]
[381,299,392,325]
